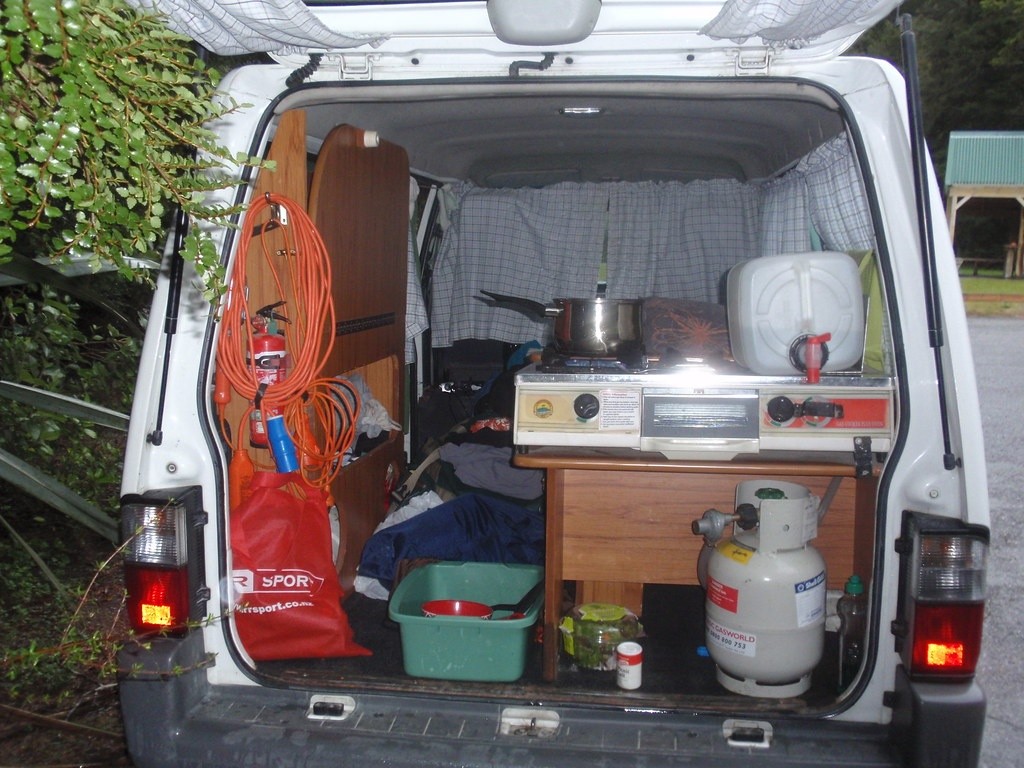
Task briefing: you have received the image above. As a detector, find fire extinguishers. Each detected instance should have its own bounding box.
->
[246,301,292,449]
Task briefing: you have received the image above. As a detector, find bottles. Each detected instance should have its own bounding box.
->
[616,642,643,689]
[829,574,868,692]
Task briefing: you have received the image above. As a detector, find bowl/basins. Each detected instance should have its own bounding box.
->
[420,600,493,621]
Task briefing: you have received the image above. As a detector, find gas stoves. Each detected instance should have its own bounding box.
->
[513,353,895,461]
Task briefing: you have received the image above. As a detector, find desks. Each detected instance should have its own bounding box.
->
[511,447,883,682]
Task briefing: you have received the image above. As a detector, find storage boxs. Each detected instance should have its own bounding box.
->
[388,560,544,682]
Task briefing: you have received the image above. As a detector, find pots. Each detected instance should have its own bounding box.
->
[480,289,643,355]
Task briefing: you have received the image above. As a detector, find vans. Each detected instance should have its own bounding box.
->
[105,0,996,768]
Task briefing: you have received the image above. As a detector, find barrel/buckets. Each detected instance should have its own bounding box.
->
[727,250,865,384]
